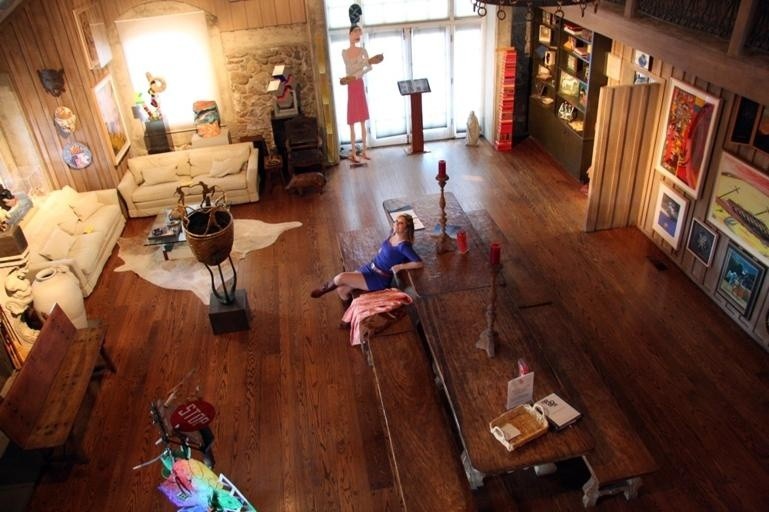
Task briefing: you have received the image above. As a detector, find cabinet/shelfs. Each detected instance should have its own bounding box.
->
[525,8,611,184]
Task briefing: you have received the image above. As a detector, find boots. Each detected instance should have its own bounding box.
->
[337,293,353,325]
[310,278,337,300]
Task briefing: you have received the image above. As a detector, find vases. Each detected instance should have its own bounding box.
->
[169,180,236,265]
[32,267,88,330]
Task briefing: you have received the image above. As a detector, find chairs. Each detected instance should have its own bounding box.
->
[283,114,328,184]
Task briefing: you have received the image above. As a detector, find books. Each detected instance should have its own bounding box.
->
[533,392,582,433]
[494,47,517,151]
[389,204,425,231]
[429,222,462,241]
[563,21,591,62]
[544,51,556,66]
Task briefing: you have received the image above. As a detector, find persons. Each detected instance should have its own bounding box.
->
[310,213,423,329]
[340,25,384,164]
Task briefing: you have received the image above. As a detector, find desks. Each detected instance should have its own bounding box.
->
[381,190,511,295]
[411,286,599,479]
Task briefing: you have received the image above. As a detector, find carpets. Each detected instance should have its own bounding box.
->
[113,212,303,306]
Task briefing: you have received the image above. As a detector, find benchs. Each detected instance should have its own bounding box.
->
[464,206,559,308]
[335,223,417,354]
[0,303,118,468]
[514,302,664,510]
[366,329,479,512]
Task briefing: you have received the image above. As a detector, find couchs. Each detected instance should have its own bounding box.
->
[117,141,259,217]
[22,184,125,297]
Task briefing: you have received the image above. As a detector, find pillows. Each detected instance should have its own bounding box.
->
[127,149,190,184]
[40,227,79,260]
[69,191,103,221]
[190,144,250,178]
[140,163,178,186]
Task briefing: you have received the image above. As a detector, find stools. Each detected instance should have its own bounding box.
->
[265,154,285,192]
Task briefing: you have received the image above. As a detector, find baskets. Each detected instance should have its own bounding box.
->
[490,403,548,452]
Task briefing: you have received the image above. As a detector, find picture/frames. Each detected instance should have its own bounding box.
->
[652,180,689,252]
[686,218,718,267]
[73,1,114,71]
[704,148,769,269]
[715,241,766,321]
[654,77,722,200]
[89,74,131,168]
